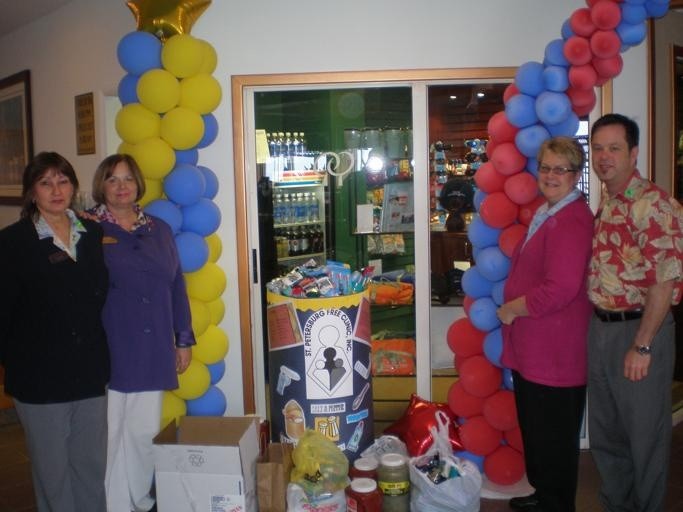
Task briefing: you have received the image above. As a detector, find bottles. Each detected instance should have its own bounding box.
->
[266,131,306,172]
[271,192,320,225]
[378,453,411,511]
[274,224,324,255]
[344,478,384,512]
[349,458,378,481]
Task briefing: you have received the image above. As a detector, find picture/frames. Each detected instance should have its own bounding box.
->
[0,68,33,206]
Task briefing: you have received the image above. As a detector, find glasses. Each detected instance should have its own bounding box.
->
[431,183,444,190]
[430,159,444,165]
[431,208,449,215]
[465,169,476,176]
[467,141,486,147]
[538,165,578,176]
[435,144,454,152]
[464,152,488,162]
[430,170,445,176]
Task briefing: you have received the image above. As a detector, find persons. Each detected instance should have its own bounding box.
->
[86,154,198,511]
[496,136,595,511]
[1,152,109,510]
[586,115,682,511]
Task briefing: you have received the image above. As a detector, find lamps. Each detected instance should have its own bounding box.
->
[256,129,272,164]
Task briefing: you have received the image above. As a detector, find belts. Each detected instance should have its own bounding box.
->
[594,306,643,323]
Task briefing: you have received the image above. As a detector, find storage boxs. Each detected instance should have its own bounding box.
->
[151,416,261,512]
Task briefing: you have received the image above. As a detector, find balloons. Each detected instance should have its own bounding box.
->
[448,217,530,485]
[472,2,668,257]
[113,0,230,430]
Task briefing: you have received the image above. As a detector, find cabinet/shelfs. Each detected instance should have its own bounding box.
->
[431,230,475,278]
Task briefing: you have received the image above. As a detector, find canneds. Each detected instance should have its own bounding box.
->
[351,457,376,476]
[380,454,412,511]
[347,476,382,512]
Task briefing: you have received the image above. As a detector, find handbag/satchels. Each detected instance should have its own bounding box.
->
[360,435,408,462]
[256,432,293,512]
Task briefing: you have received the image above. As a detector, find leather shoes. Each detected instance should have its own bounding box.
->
[510,493,543,511]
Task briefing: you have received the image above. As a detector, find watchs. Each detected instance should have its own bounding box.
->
[634,344,651,355]
